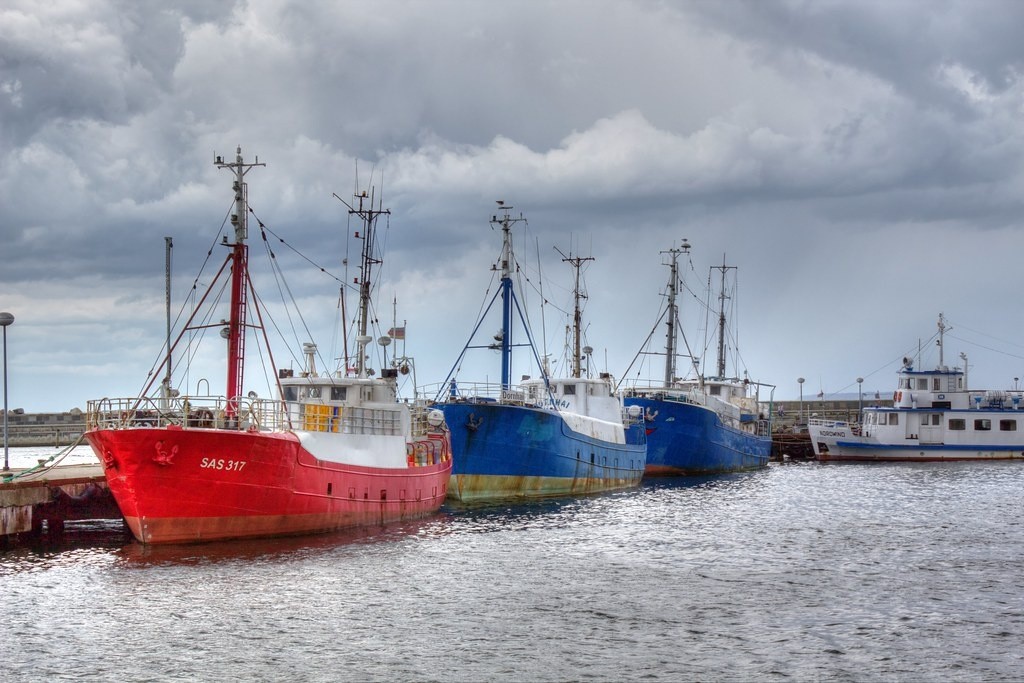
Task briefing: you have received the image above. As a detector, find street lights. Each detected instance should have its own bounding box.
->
[379,337,392,369]
[1014,377,1019,389]
[798,377,806,424]
[857,378,864,422]
[583,346,593,379]
[0,312,15,470]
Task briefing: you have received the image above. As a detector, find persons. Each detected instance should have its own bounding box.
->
[776,426,783,433]
[778,402,787,417]
[793,414,800,426]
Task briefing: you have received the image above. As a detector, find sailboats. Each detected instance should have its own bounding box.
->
[427,198,648,505]
[81,144,454,545]
[598,237,777,475]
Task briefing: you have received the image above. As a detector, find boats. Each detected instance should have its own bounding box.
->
[809,311,1024,461]
[771,425,862,461]
